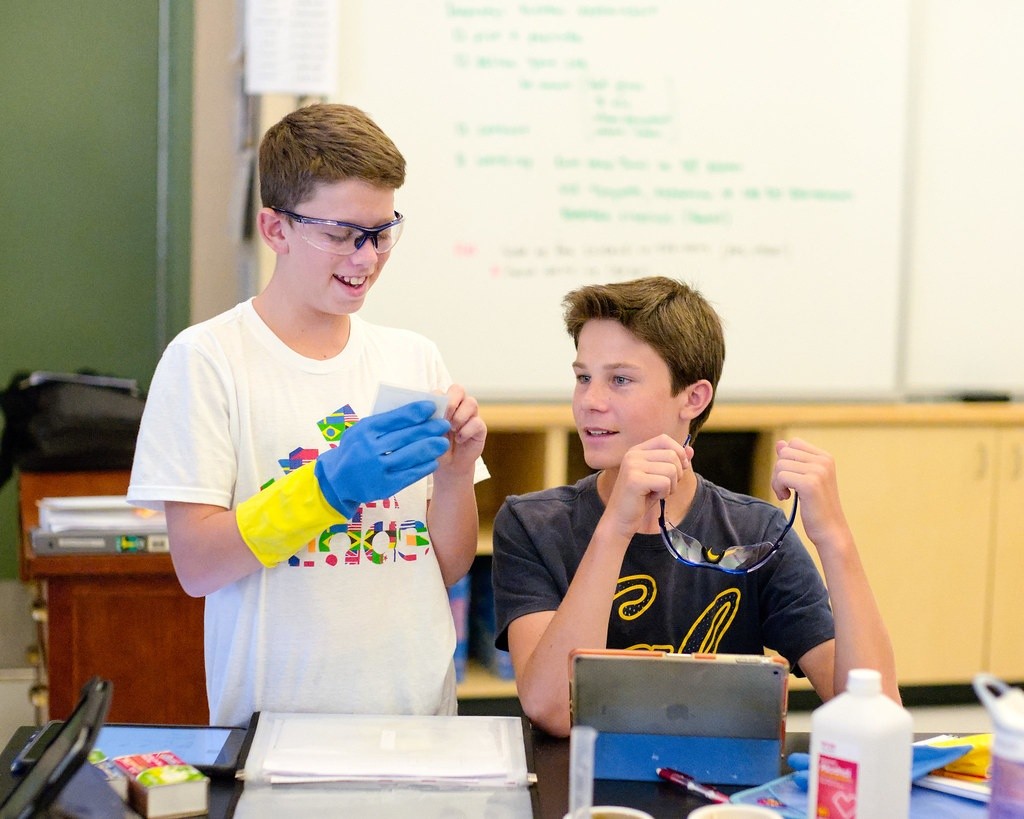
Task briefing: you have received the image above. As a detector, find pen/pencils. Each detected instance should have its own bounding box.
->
[656,767,731,804]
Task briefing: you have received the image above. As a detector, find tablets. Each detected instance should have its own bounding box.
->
[567,649,790,785]
[11,720,248,782]
[0,678,110,819]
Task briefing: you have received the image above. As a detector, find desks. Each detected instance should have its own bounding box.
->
[1,724,995,819]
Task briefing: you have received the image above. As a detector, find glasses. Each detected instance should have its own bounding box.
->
[269,203,407,257]
[657,433,801,579]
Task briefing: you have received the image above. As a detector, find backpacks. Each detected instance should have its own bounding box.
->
[0,361,147,486]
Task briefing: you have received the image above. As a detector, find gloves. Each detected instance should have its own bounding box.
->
[234,399,453,570]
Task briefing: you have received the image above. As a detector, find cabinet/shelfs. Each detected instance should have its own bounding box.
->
[449,404,777,700]
[13,469,214,729]
[773,399,1024,701]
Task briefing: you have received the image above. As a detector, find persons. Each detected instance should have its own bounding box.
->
[492,277,903,740]
[125,103,493,729]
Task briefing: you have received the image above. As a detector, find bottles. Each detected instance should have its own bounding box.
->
[973,674,1024,819]
[807,669,913,819]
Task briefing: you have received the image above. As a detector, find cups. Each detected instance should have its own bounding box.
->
[563,805,653,819]
[688,804,781,819]
